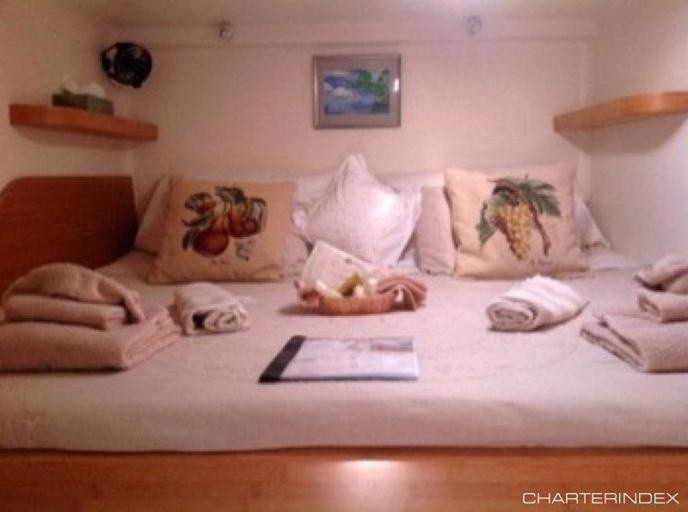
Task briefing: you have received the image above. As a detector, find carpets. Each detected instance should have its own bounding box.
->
[2,252,688,454]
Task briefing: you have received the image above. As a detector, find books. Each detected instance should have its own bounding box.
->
[257,332,420,382]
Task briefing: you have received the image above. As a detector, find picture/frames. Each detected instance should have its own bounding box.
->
[312,54,400,127]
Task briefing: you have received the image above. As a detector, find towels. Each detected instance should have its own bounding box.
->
[1,263,248,373]
[489,254,687,380]
